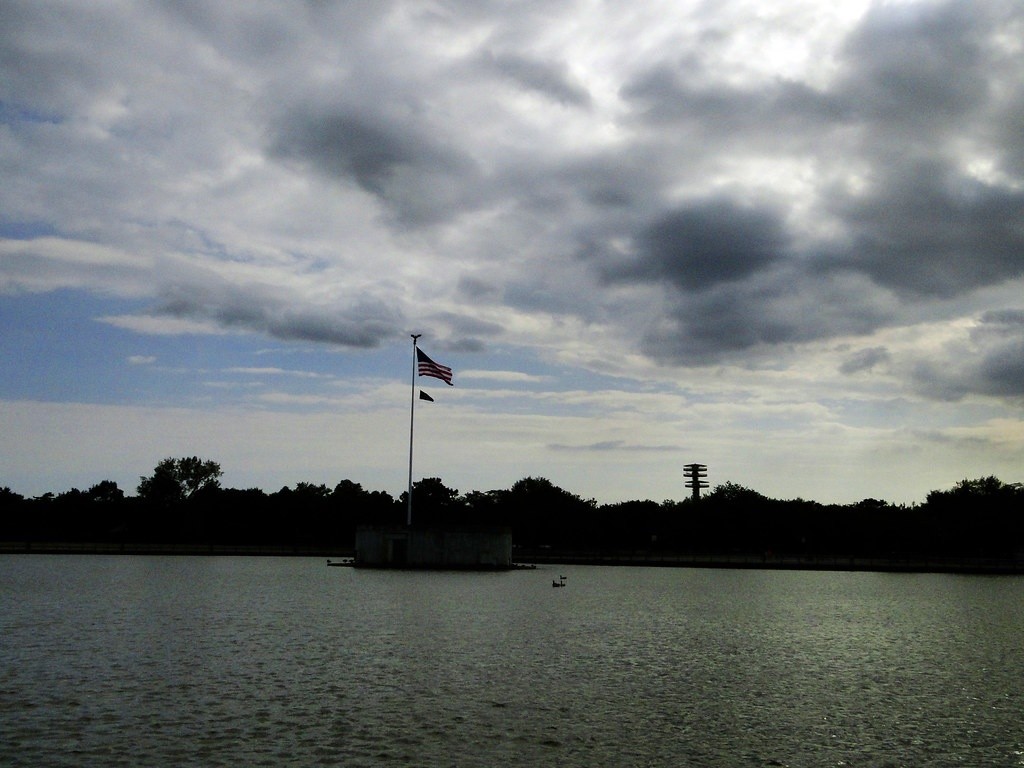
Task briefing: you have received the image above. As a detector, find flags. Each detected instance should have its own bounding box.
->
[416,346,454,387]
[419,390,434,402]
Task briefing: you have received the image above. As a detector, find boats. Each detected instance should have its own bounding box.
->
[326,524,538,571]
